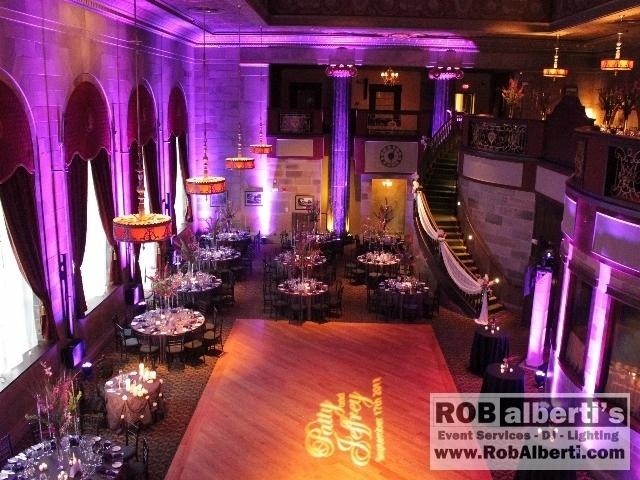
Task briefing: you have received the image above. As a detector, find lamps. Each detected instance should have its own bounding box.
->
[114,0,272,243]
[543,1,635,78]
[325,48,464,86]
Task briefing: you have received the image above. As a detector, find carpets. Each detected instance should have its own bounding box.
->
[162,320,493,479]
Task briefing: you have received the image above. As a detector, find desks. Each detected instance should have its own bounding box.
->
[475,324,576,480]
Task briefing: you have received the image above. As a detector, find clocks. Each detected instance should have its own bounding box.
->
[380,144,402,168]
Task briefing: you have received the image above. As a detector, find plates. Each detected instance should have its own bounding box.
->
[0,434,122,480]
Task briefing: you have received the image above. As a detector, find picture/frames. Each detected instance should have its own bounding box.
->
[295,195,315,210]
